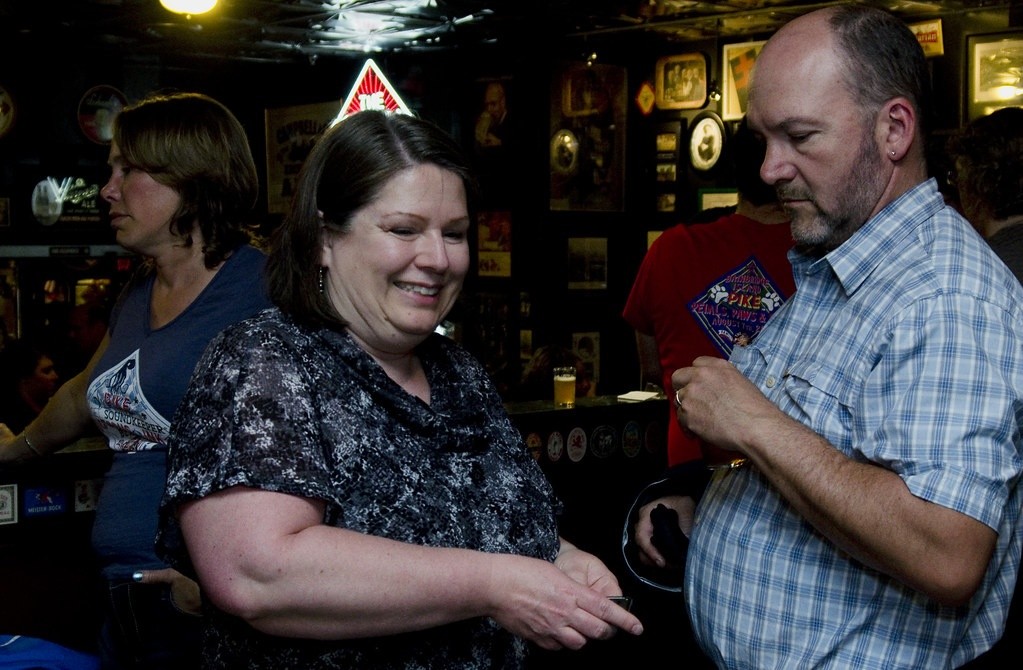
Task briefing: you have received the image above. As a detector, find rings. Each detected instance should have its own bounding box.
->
[675,390,682,406]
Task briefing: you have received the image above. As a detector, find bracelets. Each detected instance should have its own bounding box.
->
[23,428,42,455]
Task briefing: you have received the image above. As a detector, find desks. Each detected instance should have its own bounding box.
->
[499,384,671,539]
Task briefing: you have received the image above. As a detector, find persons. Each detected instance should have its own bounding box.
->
[621,1,1023,670]
[474,82,529,204]
[0,94,295,670]
[154,109,644,670]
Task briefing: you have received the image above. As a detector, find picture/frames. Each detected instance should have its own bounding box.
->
[568,327,605,390]
[959,22,1023,136]
[549,39,770,294]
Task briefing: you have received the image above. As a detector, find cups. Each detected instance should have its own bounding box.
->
[554,367,577,405]
